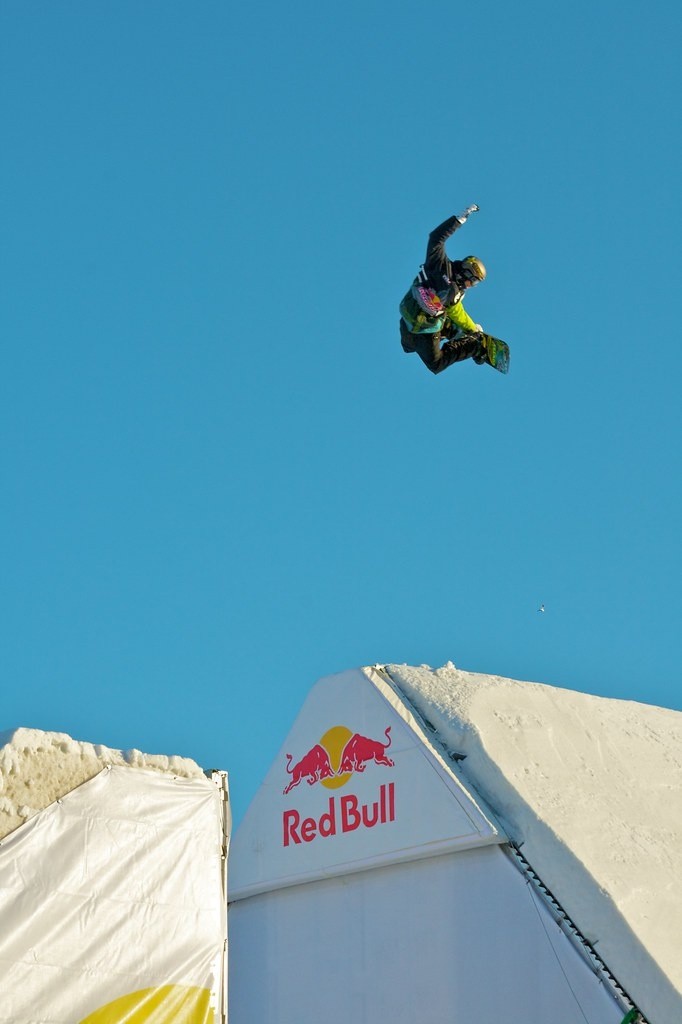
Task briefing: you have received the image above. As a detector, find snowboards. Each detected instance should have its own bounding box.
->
[443,319,511,375]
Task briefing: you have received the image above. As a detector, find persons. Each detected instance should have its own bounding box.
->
[399,203,488,375]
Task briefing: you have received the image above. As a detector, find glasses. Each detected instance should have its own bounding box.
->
[463,267,481,286]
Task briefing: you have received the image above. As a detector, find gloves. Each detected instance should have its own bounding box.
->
[475,323,483,333]
[454,203,479,224]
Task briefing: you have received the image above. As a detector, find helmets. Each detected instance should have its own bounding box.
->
[461,256,486,281]
